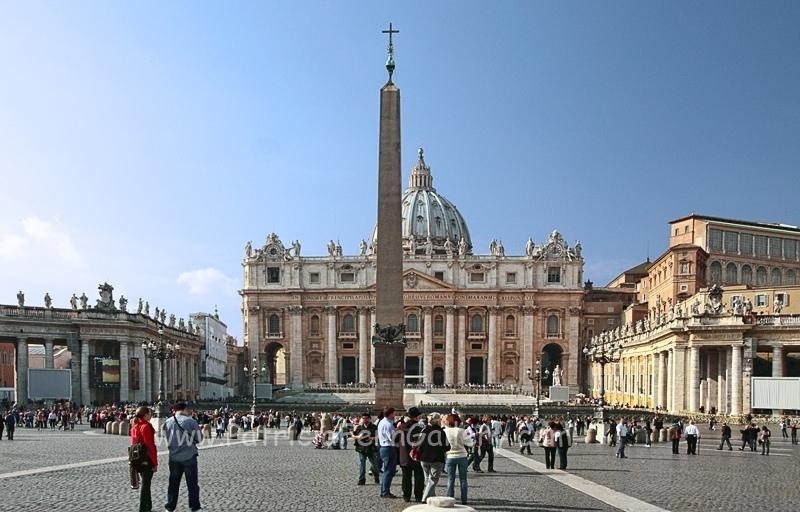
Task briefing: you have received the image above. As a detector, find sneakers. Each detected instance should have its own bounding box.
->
[716,446,771,456]
[163,500,205,512]
[672,451,698,455]
[546,466,568,472]
[613,452,628,459]
[357,469,428,504]
[471,465,497,473]
[518,450,534,457]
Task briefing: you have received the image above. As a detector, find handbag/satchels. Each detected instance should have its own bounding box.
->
[476,435,489,446]
[675,431,681,439]
[353,439,368,456]
[409,443,421,461]
[127,441,157,462]
[192,435,199,458]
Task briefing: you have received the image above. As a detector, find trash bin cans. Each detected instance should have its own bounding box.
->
[785,418,790,425]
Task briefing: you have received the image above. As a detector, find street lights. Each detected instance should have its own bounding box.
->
[526,359,550,417]
[580,328,623,436]
[142,325,181,435]
[243,354,267,419]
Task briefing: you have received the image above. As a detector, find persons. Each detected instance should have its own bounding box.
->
[137,298,201,333]
[119,295,128,312]
[79,292,88,310]
[44,292,53,308]
[17,291,24,306]
[552,364,563,384]
[70,294,79,310]
[590,284,782,347]
